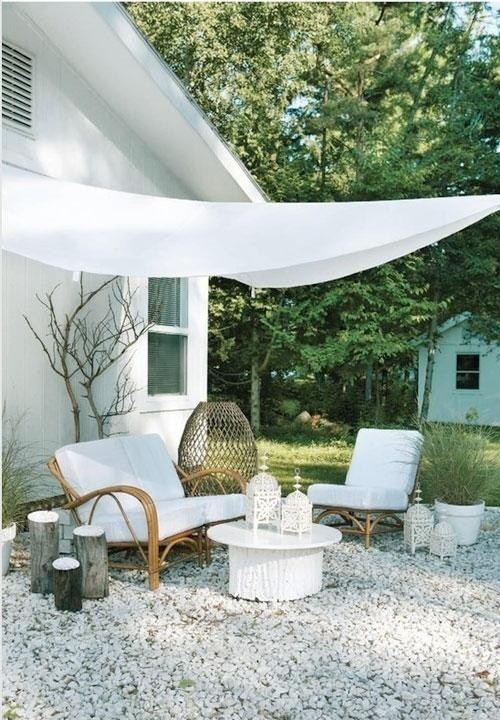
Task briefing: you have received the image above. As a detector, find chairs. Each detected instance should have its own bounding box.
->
[308,428,424,548]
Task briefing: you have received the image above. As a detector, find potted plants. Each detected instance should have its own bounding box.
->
[391,412,499,547]
[2,399,86,575]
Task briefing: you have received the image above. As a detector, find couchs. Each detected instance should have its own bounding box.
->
[45,432,248,591]
